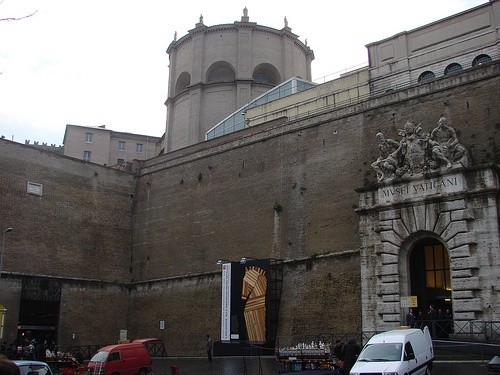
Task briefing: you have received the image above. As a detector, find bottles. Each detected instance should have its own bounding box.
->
[290,340,324,350]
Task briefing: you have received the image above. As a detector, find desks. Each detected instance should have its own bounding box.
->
[276,353,331,371]
[45,355,73,375]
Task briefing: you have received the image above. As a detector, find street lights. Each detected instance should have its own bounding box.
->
[0,226,12,276]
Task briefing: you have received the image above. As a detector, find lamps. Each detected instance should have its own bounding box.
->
[240,256,259,264]
[216,259,234,267]
[0,227,13,236]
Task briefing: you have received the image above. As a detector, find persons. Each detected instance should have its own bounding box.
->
[341,339,361,375]
[333,339,343,356]
[0,354,20,375]
[206,334,212,362]
[74,349,83,364]
[371,117,464,183]
[1,337,58,361]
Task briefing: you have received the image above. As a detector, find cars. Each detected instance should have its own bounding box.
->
[10,360,54,375]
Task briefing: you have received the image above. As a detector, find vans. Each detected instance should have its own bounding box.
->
[349,326,434,375]
[86,338,161,375]
[39,357,86,375]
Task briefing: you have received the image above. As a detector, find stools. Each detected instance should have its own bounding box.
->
[79,366,86,373]
[73,368,80,375]
